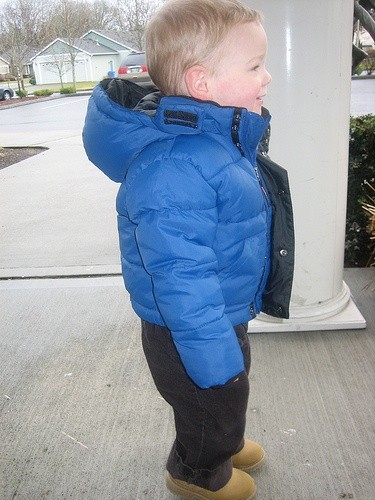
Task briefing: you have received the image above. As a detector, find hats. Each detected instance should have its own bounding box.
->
[231,439,266,471]
[167,467,257,499]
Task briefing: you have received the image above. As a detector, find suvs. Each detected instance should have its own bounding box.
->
[118,50,160,93]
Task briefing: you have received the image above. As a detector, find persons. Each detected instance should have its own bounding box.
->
[82,0,296,499]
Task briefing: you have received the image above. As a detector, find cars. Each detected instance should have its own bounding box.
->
[0,87,14,101]
